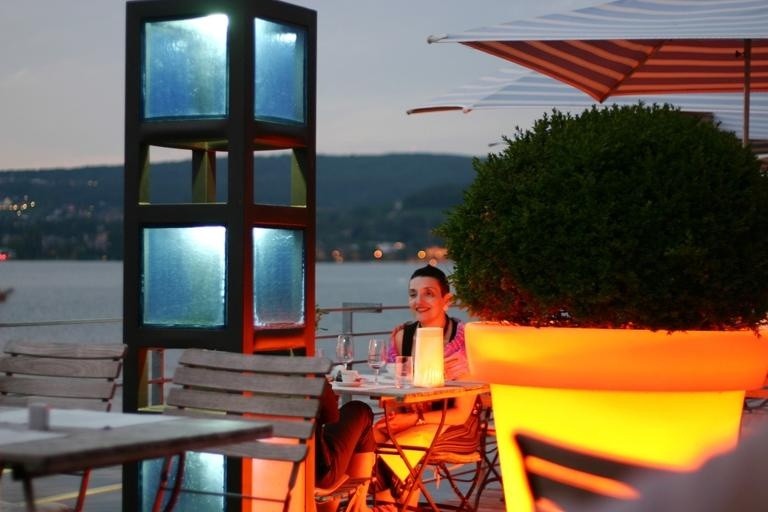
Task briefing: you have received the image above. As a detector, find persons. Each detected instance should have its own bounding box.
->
[366,261,498,512]
[260,302,376,512]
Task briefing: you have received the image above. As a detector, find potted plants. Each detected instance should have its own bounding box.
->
[426,100,767,509]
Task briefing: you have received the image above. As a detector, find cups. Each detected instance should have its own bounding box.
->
[394,356,414,390]
[339,371,357,382]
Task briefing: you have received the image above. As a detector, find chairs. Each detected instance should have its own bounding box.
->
[418,393,505,512]
[149,350,333,512]
[2,341,129,511]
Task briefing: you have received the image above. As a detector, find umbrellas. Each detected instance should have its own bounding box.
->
[425,2,767,164]
[395,54,767,165]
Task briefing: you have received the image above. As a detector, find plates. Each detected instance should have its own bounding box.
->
[336,381,362,387]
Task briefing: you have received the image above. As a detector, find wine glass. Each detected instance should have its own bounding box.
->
[368,339,386,387]
[336,333,353,369]
